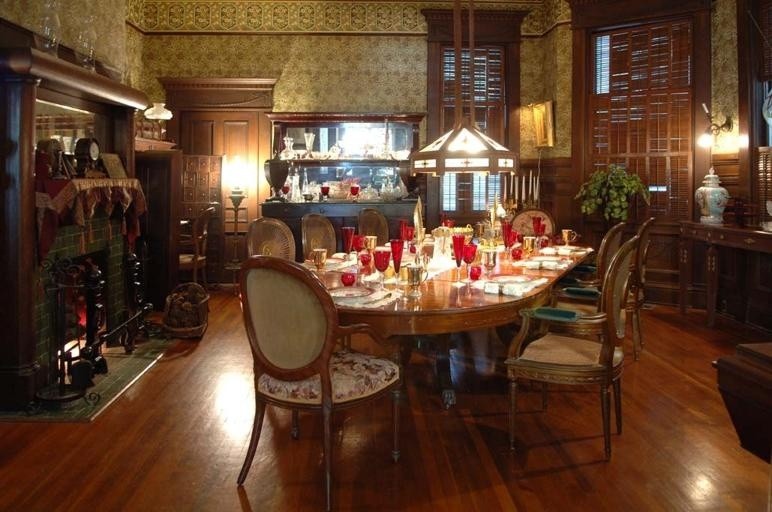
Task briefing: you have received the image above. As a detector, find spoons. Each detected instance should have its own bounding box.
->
[362,292,393,305]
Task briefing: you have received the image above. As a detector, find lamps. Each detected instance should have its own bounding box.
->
[409,2,523,176]
[694,100,733,153]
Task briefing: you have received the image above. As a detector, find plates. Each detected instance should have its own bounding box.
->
[303,256,335,267]
[327,286,375,299]
[485,244,585,286]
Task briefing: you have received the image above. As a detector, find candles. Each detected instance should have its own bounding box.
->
[500,168,539,201]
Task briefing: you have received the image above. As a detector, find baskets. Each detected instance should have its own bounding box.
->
[163,281,210,328]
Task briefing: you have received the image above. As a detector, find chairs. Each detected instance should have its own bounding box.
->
[246,206,389,260]
[238,257,400,511]
[507,209,653,460]
[175,206,215,285]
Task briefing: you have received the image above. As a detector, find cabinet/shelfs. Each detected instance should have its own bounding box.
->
[259,111,427,262]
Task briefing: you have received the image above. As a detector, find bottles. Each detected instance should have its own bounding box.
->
[281,137,294,160]
[694,171,731,229]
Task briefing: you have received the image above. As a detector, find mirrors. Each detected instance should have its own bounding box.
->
[34,98,108,153]
[279,125,412,191]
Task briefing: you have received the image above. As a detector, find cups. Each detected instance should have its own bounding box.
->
[405,267,429,296]
[310,249,327,271]
[561,230,578,247]
[304,194,314,202]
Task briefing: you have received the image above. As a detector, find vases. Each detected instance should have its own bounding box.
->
[694,164,730,223]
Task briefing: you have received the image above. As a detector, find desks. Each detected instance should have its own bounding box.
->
[673,223,772,328]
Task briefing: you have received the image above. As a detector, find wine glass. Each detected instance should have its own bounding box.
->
[304,132,315,158]
[339,214,545,282]
[350,185,360,204]
[320,187,330,201]
[282,187,289,200]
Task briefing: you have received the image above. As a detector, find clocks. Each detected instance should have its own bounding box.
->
[74,136,103,179]
